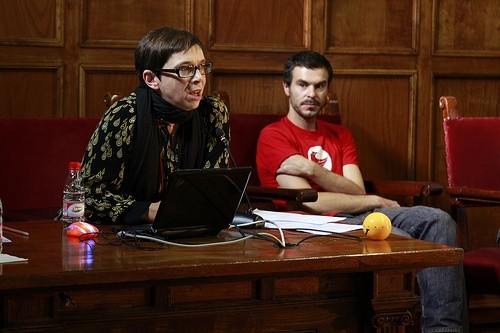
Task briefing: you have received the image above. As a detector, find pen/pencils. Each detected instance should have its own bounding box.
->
[3,226,29,236]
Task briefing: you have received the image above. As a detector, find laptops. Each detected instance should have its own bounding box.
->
[112,167,253,236]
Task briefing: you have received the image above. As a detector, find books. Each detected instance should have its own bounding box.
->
[252,208,363,236]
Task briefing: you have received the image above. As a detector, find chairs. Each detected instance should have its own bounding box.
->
[216,88,443,226]
[0,89,123,221]
[438,94,500,279]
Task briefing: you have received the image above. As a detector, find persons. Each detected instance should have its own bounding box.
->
[255,51,469,333]
[54,27,236,225]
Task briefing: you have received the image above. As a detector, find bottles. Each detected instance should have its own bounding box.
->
[61,161,86,227]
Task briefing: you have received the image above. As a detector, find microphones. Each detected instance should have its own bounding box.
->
[214,127,266,229]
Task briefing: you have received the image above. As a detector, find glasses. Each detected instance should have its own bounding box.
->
[160,62,213,78]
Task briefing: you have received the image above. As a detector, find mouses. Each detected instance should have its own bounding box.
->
[66,222,99,240]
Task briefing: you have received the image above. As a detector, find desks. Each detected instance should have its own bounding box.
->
[0,215,464,333]
